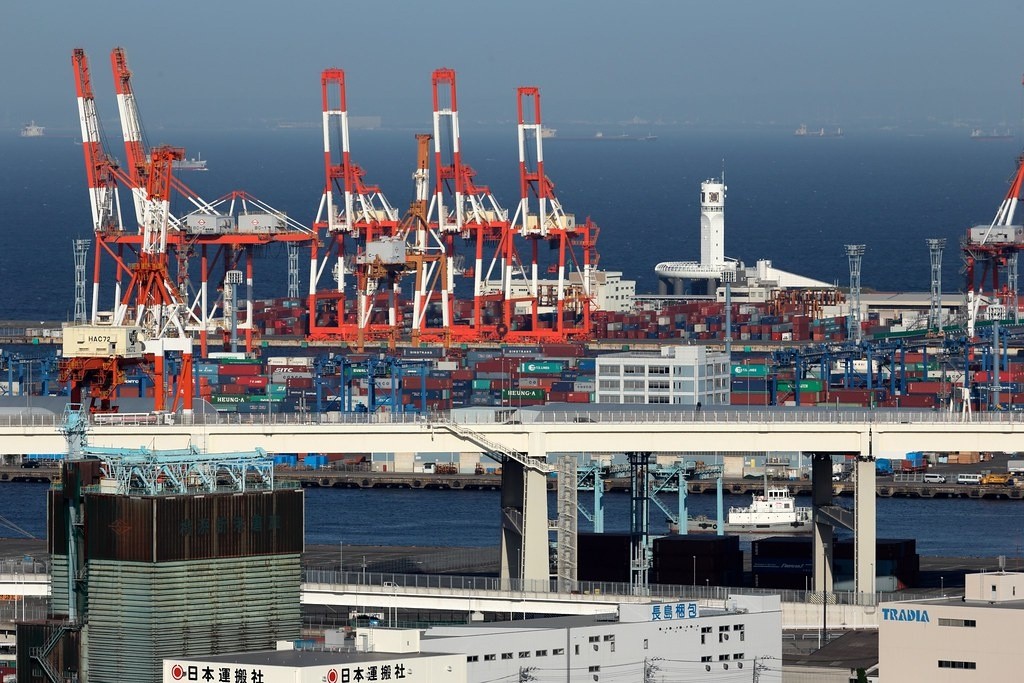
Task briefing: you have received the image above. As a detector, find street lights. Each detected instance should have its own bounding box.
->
[979,359,983,422]
[940,576,944,598]
[693,555,696,601]
[747,358,751,424]
[705,578,709,599]
[765,357,767,410]
[1008,359,1011,423]
[823,541,828,647]
[267,360,272,425]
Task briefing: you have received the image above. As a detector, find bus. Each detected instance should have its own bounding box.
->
[957,473,982,484]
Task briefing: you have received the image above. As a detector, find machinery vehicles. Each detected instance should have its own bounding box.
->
[475,463,485,475]
[980,474,1018,485]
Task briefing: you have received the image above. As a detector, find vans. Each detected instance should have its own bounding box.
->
[924,473,947,483]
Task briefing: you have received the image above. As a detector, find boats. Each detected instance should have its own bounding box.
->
[664,484,814,534]
[533,128,556,138]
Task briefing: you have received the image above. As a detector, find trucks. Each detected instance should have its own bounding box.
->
[91,411,176,426]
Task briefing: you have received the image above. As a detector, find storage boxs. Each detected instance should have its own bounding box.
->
[68,297,1024,417]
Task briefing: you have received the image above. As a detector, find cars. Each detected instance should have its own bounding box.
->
[832,475,840,481]
[21,461,40,469]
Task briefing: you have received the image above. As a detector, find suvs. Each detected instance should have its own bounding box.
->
[573,417,597,422]
[503,420,522,425]
